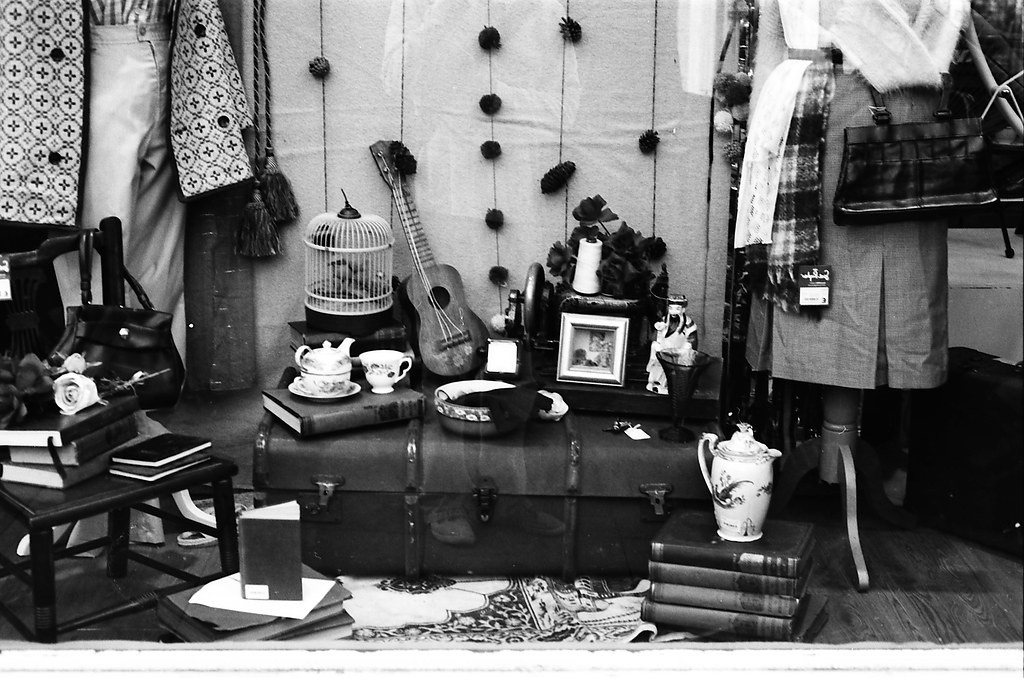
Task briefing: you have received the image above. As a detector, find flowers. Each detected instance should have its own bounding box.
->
[0,352,172,415]
[548,195,668,301]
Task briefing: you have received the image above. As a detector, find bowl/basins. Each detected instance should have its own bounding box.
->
[434,380,520,439]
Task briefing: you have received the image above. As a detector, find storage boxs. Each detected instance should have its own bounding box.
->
[253,403,729,580]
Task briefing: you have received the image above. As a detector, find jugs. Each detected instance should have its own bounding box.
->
[294,337,356,395]
[697,422,783,544]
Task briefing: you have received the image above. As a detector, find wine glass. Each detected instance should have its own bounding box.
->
[655,348,711,442]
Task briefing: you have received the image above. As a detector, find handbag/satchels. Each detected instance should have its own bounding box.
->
[831,117,998,224]
[47,228,183,416]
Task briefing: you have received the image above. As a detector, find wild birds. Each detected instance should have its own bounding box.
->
[328,259,369,284]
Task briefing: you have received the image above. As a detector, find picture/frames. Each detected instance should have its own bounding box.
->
[556,311,630,387]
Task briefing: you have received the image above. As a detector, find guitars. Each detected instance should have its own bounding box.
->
[369,139,491,377]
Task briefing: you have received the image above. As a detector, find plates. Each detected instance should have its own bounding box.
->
[287,381,361,398]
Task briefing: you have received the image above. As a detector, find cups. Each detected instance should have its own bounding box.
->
[359,350,413,395]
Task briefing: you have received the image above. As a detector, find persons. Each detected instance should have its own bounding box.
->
[734,0,973,389]
[0,0,254,327]
[645,294,698,395]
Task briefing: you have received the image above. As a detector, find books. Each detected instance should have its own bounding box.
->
[640,510,829,643]
[0,395,213,490]
[154,500,355,644]
[262,318,427,438]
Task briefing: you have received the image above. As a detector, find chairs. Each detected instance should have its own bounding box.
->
[0,214,238,644]
